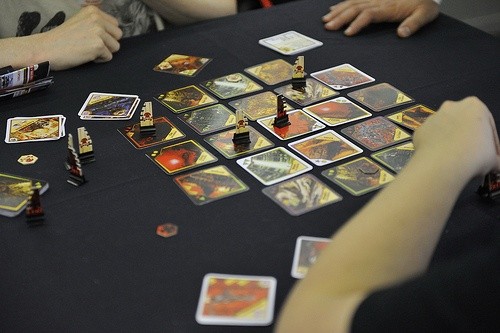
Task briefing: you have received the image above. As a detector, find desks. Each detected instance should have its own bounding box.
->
[2,0,499,333]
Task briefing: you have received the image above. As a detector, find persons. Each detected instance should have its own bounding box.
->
[1,0,239,74]
[321,1,500,37]
[273,96,500,332]
